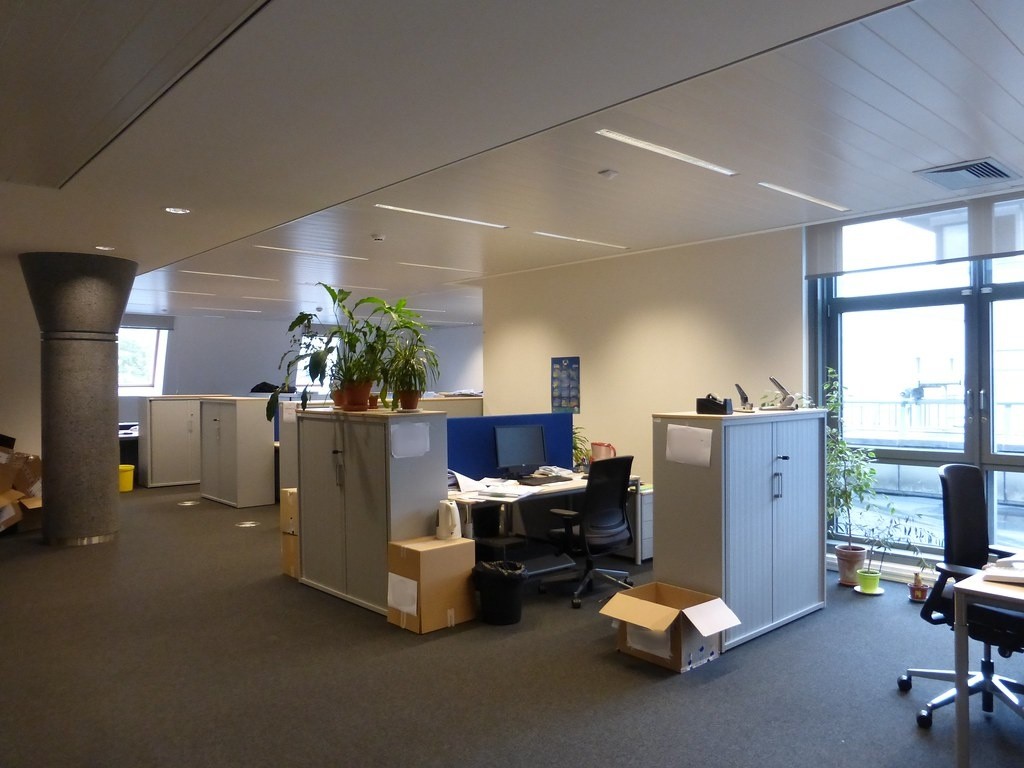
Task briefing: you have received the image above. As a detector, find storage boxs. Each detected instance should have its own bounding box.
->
[280,531,298,580]
[280,487,299,536]
[386,536,476,635]
[600,582,742,674]
[0,434,44,535]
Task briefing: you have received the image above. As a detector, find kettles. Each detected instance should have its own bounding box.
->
[436,500,462,540]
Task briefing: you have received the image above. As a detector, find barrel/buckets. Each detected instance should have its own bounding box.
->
[118,464,135,492]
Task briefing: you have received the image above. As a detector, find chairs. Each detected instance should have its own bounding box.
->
[537,454,635,608]
[897,462,1024,728]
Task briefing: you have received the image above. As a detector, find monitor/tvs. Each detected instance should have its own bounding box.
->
[492,424,547,480]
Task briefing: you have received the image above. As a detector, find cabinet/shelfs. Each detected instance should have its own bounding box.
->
[138,393,484,618]
[651,408,826,653]
[616,486,654,561]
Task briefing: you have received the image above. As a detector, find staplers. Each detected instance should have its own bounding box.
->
[760,377,799,411]
[732,384,754,413]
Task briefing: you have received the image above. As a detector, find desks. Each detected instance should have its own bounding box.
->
[952,554,1024,768]
[449,476,642,568]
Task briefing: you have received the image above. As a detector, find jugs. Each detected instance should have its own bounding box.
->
[591,442,616,461]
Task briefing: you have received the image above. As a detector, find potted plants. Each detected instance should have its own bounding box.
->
[816,367,945,601]
[265,283,440,411]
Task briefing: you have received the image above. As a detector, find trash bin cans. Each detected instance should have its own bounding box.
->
[474,561,529,624]
[119,464,135,494]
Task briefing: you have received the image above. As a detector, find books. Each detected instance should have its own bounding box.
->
[478,486,520,497]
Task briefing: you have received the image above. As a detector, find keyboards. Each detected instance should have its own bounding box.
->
[517,475,573,486]
